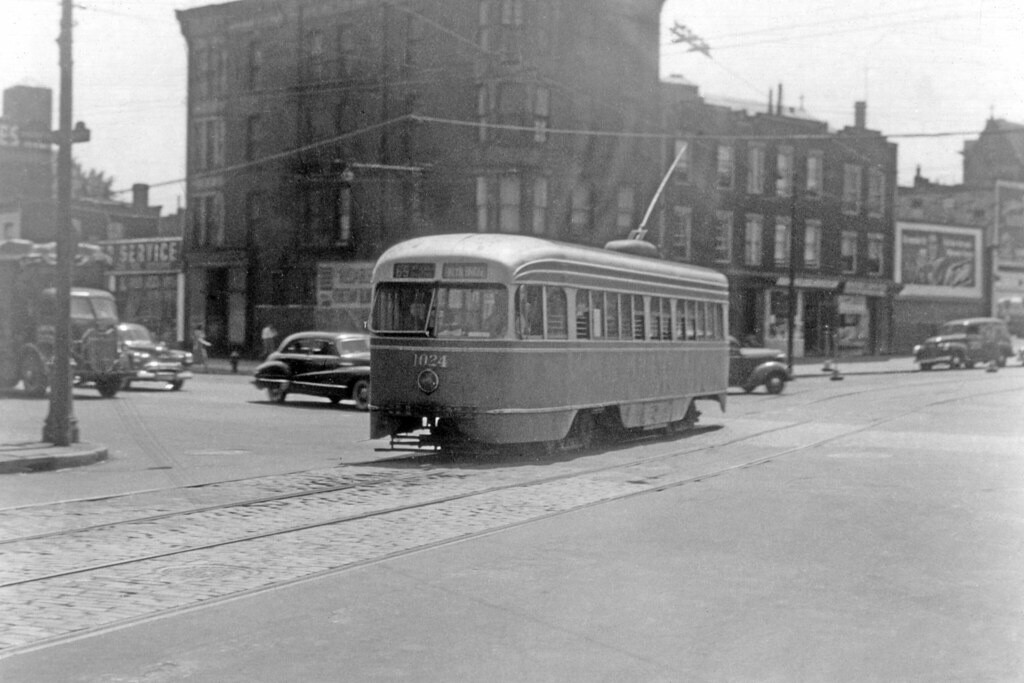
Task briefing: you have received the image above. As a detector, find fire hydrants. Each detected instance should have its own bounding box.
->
[231,351,241,372]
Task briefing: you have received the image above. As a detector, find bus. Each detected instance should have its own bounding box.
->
[366,233,732,459]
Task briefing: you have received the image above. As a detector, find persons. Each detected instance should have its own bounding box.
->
[191,322,211,374]
[260,322,279,358]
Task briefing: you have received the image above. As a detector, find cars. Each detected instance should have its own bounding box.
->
[652,331,793,394]
[117,321,193,390]
[252,329,372,410]
[912,316,1014,371]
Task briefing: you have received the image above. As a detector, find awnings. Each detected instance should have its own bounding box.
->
[836,274,902,300]
[754,271,842,294]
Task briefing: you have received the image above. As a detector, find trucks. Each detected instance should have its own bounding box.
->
[0,239,136,397]
